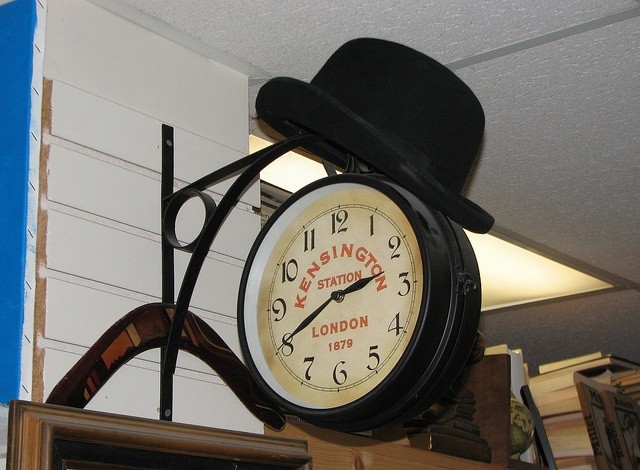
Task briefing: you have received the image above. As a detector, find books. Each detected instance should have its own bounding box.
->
[483,343,640,470]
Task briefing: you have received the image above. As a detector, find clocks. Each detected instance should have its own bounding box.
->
[237,170,481,445]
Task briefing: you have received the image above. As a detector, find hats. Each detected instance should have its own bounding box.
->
[255,38,494,234]
